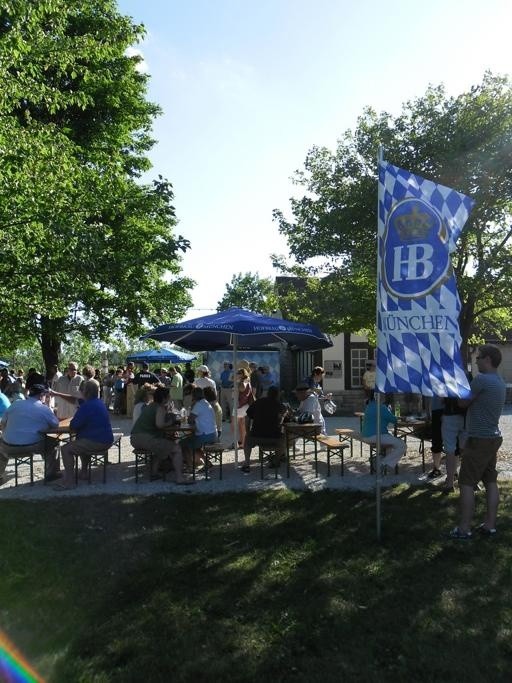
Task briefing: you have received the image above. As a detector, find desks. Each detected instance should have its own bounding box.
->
[163,422,195,480]
[43,417,79,486]
[355,411,426,474]
[283,420,321,478]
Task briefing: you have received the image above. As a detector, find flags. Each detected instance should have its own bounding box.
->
[375,158,477,402]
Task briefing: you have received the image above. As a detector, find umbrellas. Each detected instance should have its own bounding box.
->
[137,307,334,469]
[126,345,200,383]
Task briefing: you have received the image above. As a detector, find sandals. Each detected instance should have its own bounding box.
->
[53,483,77,492]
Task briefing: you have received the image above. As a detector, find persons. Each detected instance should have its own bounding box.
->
[226,368,247,449]
[191,364,218,397]
[0,368,12,397]
[361,397,407,473]
[4,383,23,404]
[129,386,198,485]
[0,383,64,482]
[168,366,182,408]
[101,371,116,411]
[427,397,460,482]
[0,389,12,422]
[203,385,223,438]
[185,362,197,383]
[178,385,220,474]
[14,380,26,400]
[57,361,84,418]
[47,381,114,491]
[59,366,69,380]
[431,372,474,493]
[298,366,333,400]
[361,362,376,404]
[258,363,266,381]
[112,368,124,386]
[292,383,326,436]
[13,369,26,380]
[240,385,287,469]
[50,364,62,390]
[220,359,235,423]
[159,368,171,384]
[117,362,135,413]
[79,364,101,399]
[133,361,164,387]
[438,344,510,541]
[248,360,257,377]
[26,367,47,396]
[133,387,149,426]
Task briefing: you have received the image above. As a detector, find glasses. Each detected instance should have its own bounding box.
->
[475,357,483,360]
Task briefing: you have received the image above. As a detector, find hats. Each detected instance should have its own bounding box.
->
[196,365,210,373]
[27,383,48,397]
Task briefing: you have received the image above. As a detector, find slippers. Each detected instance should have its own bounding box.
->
[177,478,197,486]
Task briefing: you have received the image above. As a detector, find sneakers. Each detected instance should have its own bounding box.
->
[442,527,472,540]
[428,468,441,479]
[475,523,497,537]
[45,471,63,482]
[438,487,454,493]
[240,463,250,473]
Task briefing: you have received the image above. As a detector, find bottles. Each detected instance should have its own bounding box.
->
[395,401,400,416]
[180,407,188,418]
[388,404,392,412]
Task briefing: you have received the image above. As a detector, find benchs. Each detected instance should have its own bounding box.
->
[307,433,349,476]
[88,432,123,484]
[204,439,224,480]
[393,428,432,455]
[335,429,387,474]
[133,448,153,483]
[258,441,279,479]
[8,452,37,487]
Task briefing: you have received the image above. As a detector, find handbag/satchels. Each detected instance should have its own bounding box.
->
[247,391,257,406]
[323,399,336,415]
